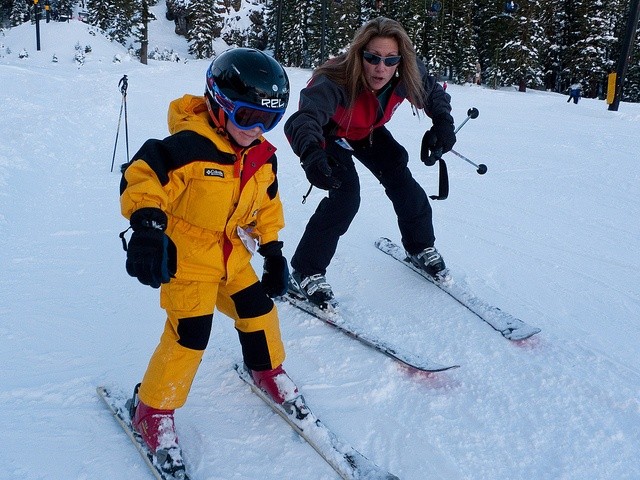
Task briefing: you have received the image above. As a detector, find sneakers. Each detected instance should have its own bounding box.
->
[243,364,299,403]
[405,245,446,275]
[290,270,336,305]
[129,383,178,453]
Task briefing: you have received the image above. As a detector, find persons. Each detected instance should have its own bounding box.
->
[567,79,581,104]
[285,16,456,310]
[120,47,299,454]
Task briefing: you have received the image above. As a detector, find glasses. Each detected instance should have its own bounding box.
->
[207,62,288,132]
[361,48,401,67]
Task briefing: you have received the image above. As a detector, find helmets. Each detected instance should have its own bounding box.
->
[207,47,289,132]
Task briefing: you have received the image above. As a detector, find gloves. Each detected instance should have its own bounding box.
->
[301,143,341,190]
[257,241,288,298]
[119,207,177,288]
[430,115,456,152]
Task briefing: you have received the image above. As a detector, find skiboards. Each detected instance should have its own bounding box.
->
[274,238,542,373]
[97,360,402,480]
